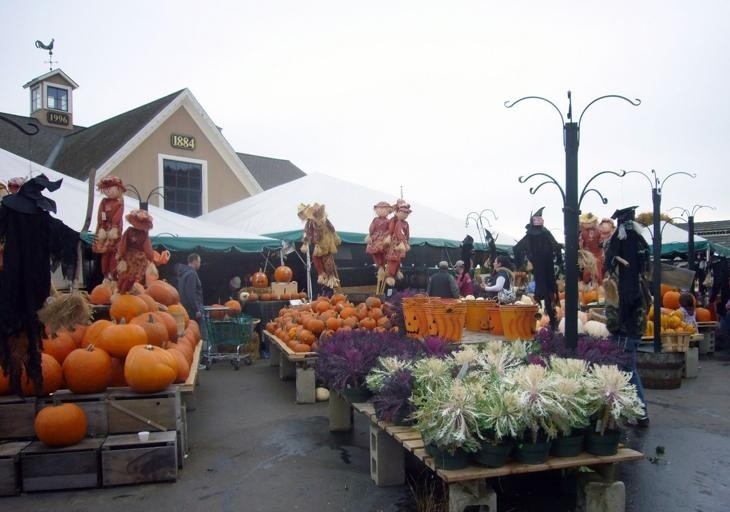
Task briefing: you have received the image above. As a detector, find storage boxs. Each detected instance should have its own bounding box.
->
[0,387,183,494]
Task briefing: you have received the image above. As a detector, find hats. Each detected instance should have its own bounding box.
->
[439,260,448,267]
[453,260,464,266]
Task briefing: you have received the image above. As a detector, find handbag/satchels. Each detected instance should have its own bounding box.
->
[498,288,515,303]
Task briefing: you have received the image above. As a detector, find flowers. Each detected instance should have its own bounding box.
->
[310,324,650,469]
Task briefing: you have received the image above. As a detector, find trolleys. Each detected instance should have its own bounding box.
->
[203,306,261,372]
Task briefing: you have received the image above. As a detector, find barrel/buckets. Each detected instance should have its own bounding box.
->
[227,317,260,336]
[402,292,538,344]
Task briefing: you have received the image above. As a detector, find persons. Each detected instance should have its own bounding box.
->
[512,205,563,315]
[170,251,208,373]
[222,275,243,303]
[479,253,512,304]
[612,278,651,428]
[364,201,413,288]
[0,174,68,396]
[577,205,650,349]
[424,260,475,298]
[112,209,154,296]
[298,203,340,289]
[89,173,128,276]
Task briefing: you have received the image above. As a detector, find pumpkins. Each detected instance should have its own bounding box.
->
[210,304,226,320]
[34,396,88,446]
[457,290,610,338]
[249,288,399,352]
[224,300,241,317]
[0,279,200,395]
[252,268,268,287]
[646,284,710,336]
[274,261,292,282]
